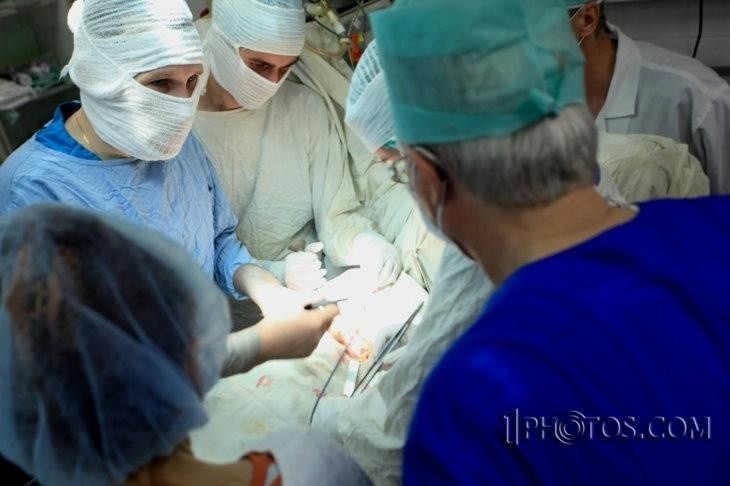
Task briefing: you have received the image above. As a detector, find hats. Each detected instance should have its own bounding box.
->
[368,0,587,145]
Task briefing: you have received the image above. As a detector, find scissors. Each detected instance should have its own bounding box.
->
[305,298,348,309]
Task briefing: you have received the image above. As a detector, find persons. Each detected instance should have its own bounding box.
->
[0,2,730,486]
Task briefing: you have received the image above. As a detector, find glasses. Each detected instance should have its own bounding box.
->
[388,146,445,185]
